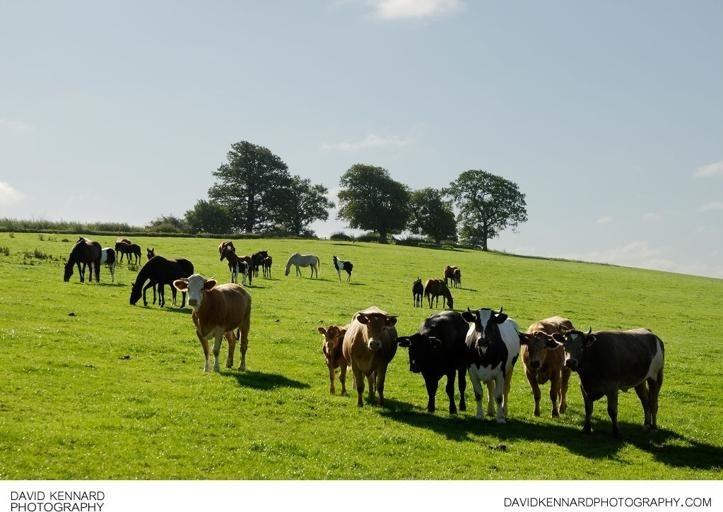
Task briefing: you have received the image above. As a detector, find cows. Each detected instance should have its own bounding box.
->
[341,305,398,408]
[397,309,469,417]
[316,323,353,396]
[551,322,665,438]
[520,315,576,419]
[174,273,251,373]
[462,306,522,425]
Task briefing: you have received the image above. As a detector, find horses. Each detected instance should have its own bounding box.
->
[218,241,272,286]
[114,238,141,266]
[412,276,423,308]
[76,236,117,283]
[284,252,319,280]
[443,265,462,289]
[64,236,102,284]
[423,279,454,310]
[333,254,354,283]
[146,248,175,305]
[129,255,194,309]
